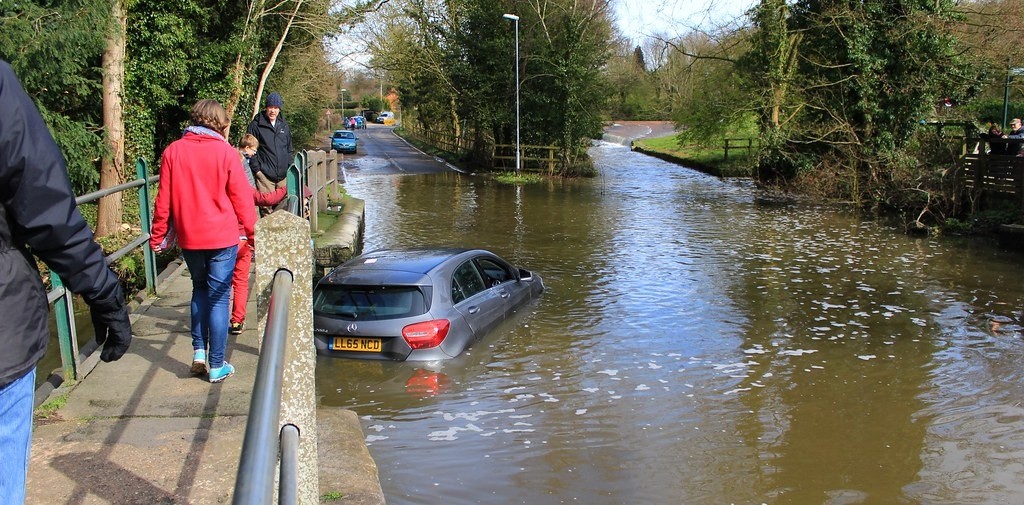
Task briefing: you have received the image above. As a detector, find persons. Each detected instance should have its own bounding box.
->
[344,117,349,131]
[0,59,132,505]
[152,91,293,381]
[350,117,362,131]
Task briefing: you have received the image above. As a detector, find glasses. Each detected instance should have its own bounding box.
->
[248,146,257,152]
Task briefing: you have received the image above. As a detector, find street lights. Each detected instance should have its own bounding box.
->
[503,12,521,171]
[340,88,346,122]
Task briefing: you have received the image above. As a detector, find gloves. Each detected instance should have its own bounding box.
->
[88,286,131,363]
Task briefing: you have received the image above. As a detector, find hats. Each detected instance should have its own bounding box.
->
[265,91,282,110]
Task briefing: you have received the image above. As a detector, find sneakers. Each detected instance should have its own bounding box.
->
[232,322,244,333]
[209,361,235,383]
[189,349,207,375]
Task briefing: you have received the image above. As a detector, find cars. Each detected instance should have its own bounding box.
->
[321,297,543,399]
[376,112,394,123]
[329,130,360,154]
[344,115,367,130]
[314,246,545,363]
